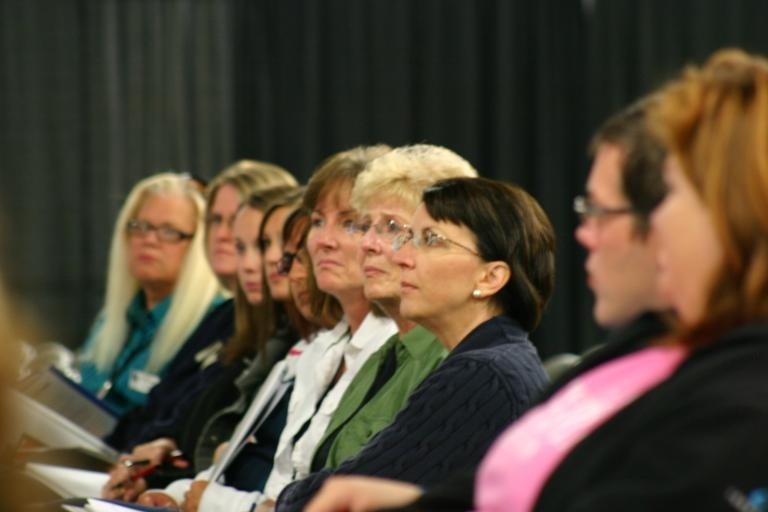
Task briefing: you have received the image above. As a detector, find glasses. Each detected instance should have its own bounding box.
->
[274,245,310,275]
[125,218,194,242]
[351,215,408,234]
[398,224,481,256]
[573,196,633,217]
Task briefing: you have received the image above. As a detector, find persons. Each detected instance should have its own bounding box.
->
[304,92,673,510]
[273,177,555,512]
[306,146,483,475]
[135,209,337,512]
[473,50,768,511]
[252,142,392,512]
[104,162,299,454]
[194,192,305,474]
[101,184,297,503]
[73,171,229,415]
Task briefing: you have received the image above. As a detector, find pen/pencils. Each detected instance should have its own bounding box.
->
[110,444,186,491]
[121,454,190,469]
